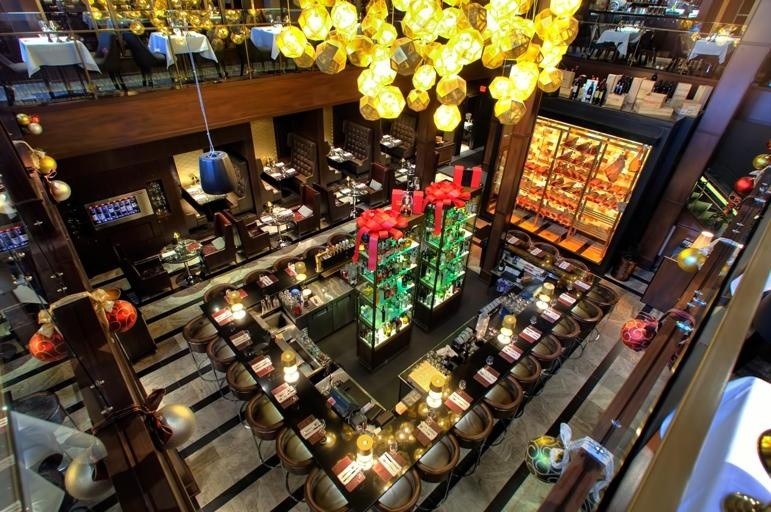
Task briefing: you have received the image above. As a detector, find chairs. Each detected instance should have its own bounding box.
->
[567,20,734,76]
[0,21,288,102]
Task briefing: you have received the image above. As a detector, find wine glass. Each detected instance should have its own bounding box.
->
[529,315,538,329]
[458,380,467,395]
[486,355,494,369]
[549,297,558,310]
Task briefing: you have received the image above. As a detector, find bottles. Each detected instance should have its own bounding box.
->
[560,43,698,116]
[351,208,425,348]
[260,288,312,316]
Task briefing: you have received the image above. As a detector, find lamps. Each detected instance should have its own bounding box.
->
[276,0,586,134]
[179,30,239,196]
